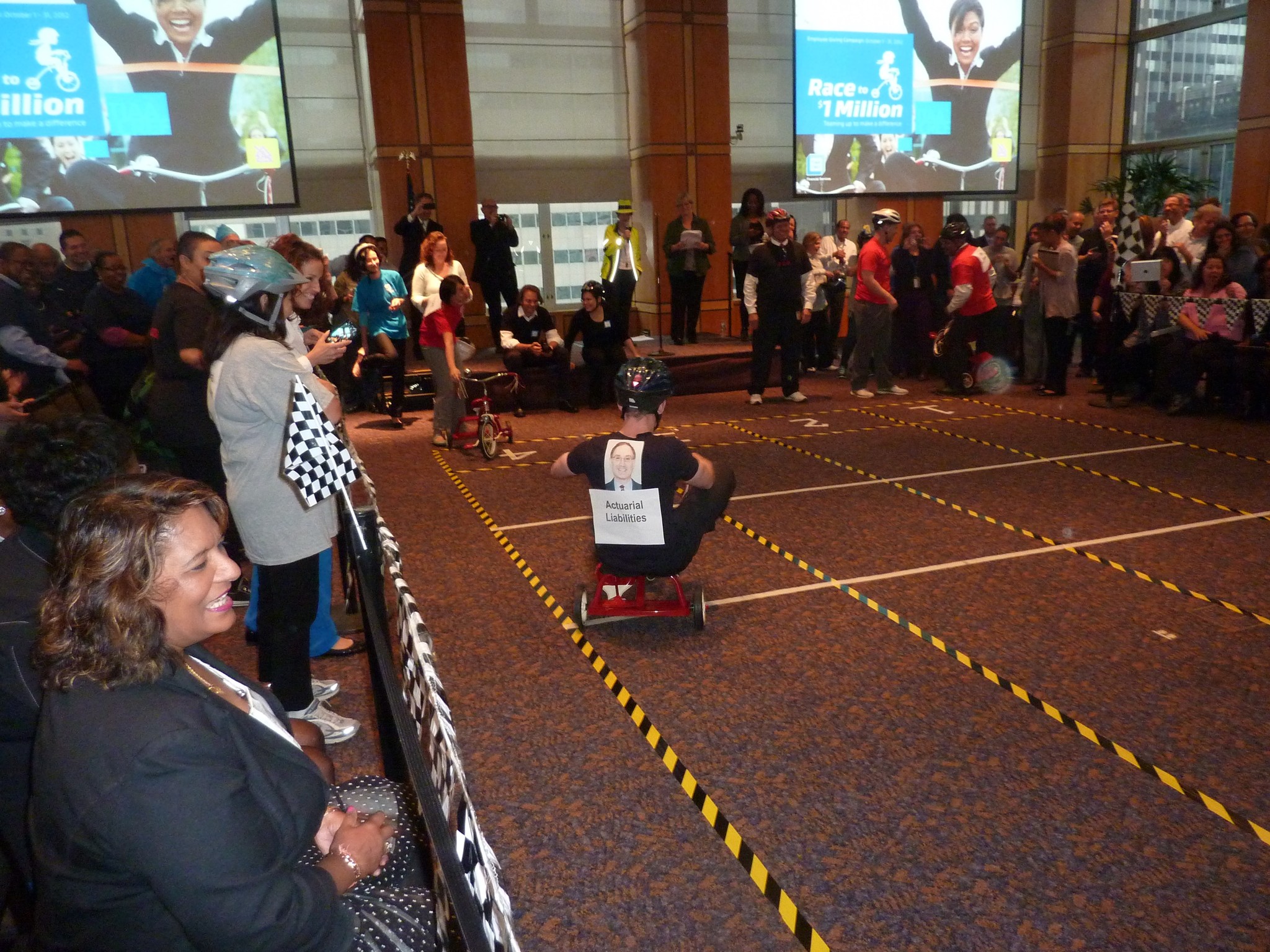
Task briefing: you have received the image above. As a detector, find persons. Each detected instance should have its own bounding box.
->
[410,231,473,341]
[0,0,276,212]
[204,244,359,745]
[1014,193,1270,419]
[0,231,409,659]
[605,442,641,491]
[498,285,579,416]
[394,193,466,364]
[17,473,437,952]
[551,358,736,579]
[795,0,1022,195]
[0,422,335,931]
[418,275,477,444]
[562,190,714,417]
[728,187,1021,405]
[469,197,521,354]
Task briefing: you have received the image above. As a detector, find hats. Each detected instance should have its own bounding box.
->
[616,200,636,213]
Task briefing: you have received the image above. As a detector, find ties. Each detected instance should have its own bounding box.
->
[620,485,625,491]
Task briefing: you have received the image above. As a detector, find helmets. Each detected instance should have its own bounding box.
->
[871,208,901,224]
[614,357,671,393]
[940,222,970,240]
[766,208,790,227]
[581,280,606,297]
[203,245,311,303]
[354,243,376,261]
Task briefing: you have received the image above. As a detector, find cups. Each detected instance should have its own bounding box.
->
[626,222,633,231]
[349,288,355,302]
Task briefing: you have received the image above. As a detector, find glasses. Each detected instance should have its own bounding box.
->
[612,455,633,462]
[782,246,787,263]
[483,205,498,210]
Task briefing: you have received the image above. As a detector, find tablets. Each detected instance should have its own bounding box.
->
[1129,259,1163,283]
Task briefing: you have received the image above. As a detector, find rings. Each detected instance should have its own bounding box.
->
[386,843,390,854]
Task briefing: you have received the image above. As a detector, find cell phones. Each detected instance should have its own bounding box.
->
[497,214,505,221]
[326,318,362,344]
[423,202,436,210]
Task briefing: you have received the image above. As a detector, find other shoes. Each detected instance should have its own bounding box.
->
[750,353,1064,405]
[559,399,579,413]
[352,356,362,378]
[392,416,402,424]
[688,337,698,344]
[590,400,599,409]
[516,408,525,416]
[433,430,447,445]
[674,338,683,345]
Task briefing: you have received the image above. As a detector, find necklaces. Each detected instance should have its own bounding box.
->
[182,659,223,695]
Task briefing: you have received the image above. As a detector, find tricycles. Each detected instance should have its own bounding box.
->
[446,372,518,461]
[574,564,712,631]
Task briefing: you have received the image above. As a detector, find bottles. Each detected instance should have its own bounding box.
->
[762,232,769,243]
[914,275,920,288]
[720,323,727,338]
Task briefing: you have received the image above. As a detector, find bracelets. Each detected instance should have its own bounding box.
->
[325,848,361,886]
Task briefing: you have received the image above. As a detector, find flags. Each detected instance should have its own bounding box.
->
[1110,171,1145,290]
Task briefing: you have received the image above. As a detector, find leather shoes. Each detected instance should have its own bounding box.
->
[245,627,259,644]
[318,638,367,656]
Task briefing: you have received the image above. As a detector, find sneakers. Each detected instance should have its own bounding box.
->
[227,577,251,606]
[285,697,361,744]
[311,674,339,701]
